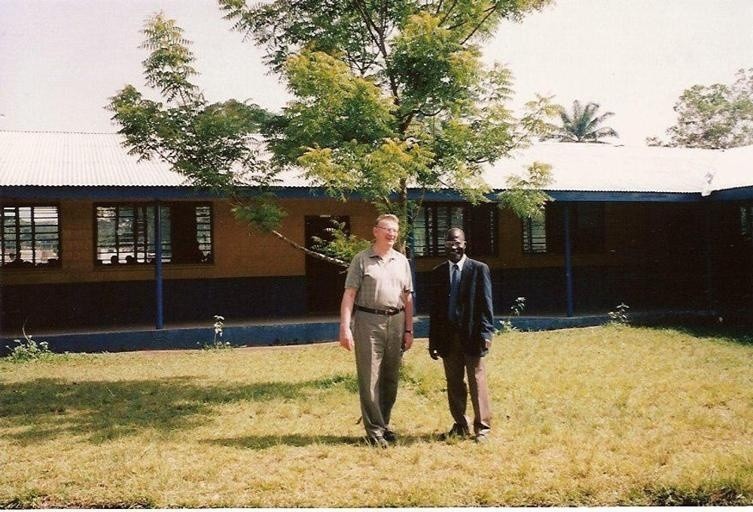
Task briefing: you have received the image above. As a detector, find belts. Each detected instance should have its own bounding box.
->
[354,303,404,316]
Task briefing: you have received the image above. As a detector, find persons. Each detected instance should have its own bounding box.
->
[110,255,138,265]
[338,213,494,449]
[196,241,212,264]
[8,251,23,264]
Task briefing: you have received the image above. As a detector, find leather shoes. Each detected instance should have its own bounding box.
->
[436,422,490,444]
[370,430,396,448]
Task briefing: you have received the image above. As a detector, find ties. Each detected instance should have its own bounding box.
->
[449,265,460,321]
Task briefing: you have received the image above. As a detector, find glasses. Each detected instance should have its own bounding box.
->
[376,225,399,232]
